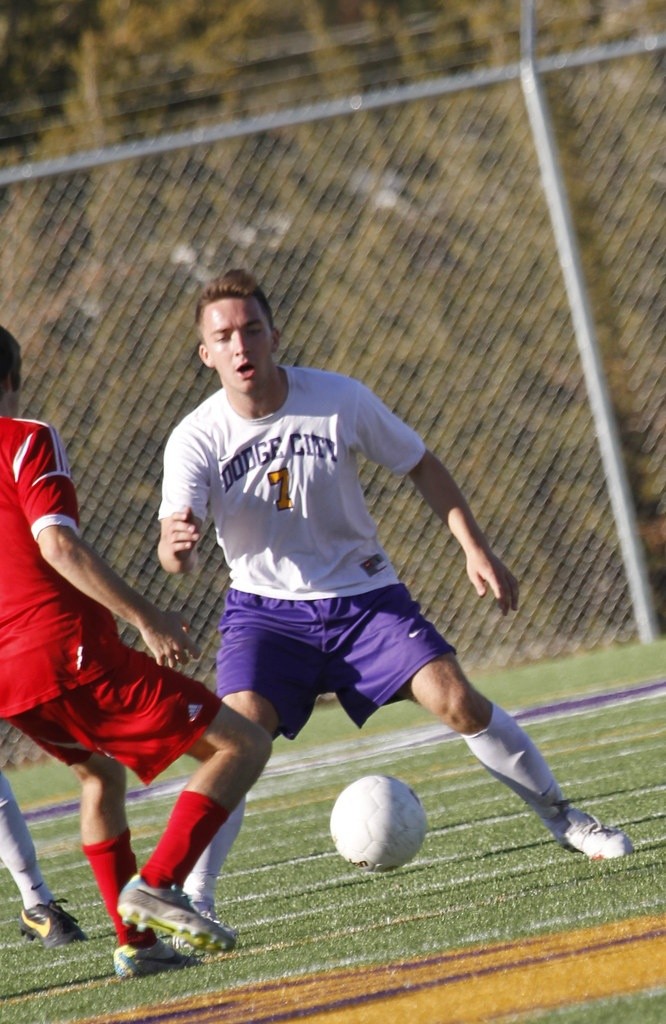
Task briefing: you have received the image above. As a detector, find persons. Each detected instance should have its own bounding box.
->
[0,770,89,949]
[153,269,633,950]
[0,326,273,977]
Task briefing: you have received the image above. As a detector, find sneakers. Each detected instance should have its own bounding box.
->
[18,899,86,949]
[555,808,635,858]
[173,893,216,954]
[113,939,203,977]
[116,872,236,958]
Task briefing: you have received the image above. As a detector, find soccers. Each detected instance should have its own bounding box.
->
[330,773,428,872]
